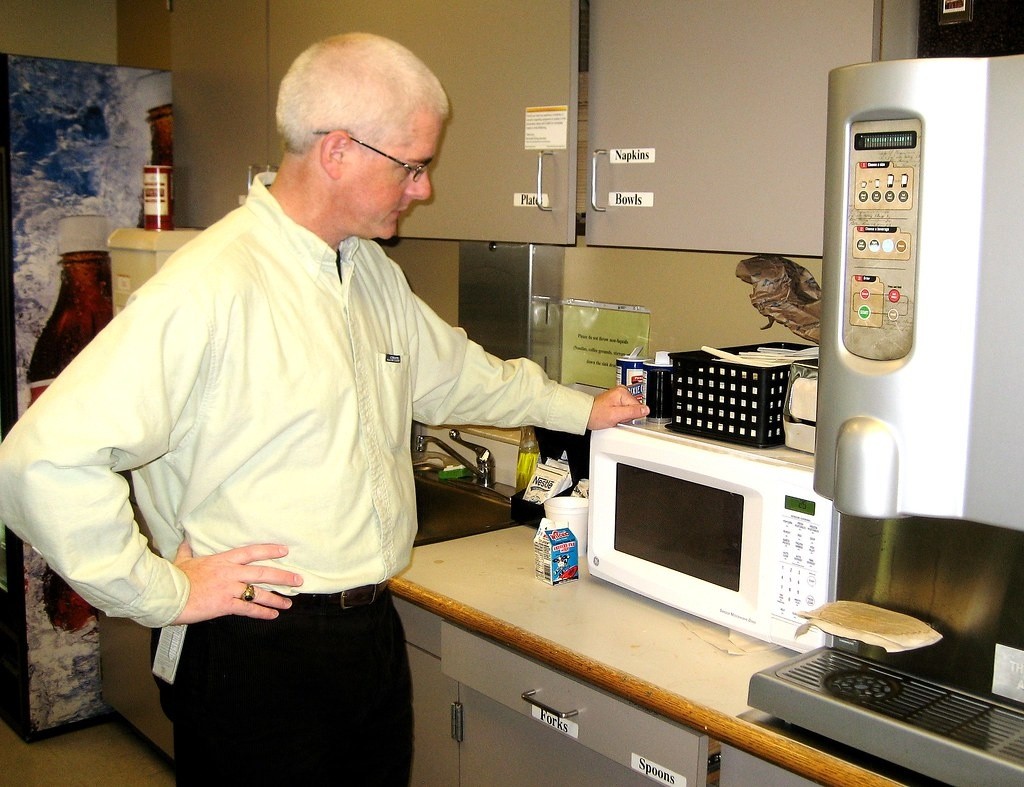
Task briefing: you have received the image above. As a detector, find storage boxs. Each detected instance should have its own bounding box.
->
[664,341,819,449]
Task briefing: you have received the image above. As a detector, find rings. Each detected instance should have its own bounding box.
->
[241,583,255,601]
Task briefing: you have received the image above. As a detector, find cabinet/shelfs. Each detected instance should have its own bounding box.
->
[171,0,918,257]
[381,600,821,787]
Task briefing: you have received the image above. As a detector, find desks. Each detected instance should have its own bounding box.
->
[383,529,910,787]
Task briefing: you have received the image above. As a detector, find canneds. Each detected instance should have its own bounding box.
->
[143,164,173,229]
[616,346,674,424]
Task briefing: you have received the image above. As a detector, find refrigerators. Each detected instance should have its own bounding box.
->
[0,50,174,744]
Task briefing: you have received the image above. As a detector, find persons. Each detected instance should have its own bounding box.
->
[0,33,651,787]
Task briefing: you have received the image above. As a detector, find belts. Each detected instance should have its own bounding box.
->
[272,578,389,610]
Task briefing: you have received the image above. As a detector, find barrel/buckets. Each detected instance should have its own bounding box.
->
[615,346,674,424]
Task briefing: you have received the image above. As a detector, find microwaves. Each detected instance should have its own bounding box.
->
[587,420,841,656]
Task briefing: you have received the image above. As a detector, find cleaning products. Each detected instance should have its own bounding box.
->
[515,425,540,493]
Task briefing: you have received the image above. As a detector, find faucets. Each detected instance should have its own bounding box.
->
[415,429,496,488]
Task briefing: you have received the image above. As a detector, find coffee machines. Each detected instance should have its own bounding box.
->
[746,52,1024,787]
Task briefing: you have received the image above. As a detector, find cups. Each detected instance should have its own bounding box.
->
[143,166,173,232]
[247,163,279,189]
[543,496,589,557]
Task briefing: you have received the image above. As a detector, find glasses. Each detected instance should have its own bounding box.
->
[313,131,433,183]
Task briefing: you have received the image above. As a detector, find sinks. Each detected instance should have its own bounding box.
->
[413,470,522,547]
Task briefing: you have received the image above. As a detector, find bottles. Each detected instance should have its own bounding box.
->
[515,425,539,494]
[25,214,113,635]
[136,103,173,229]
